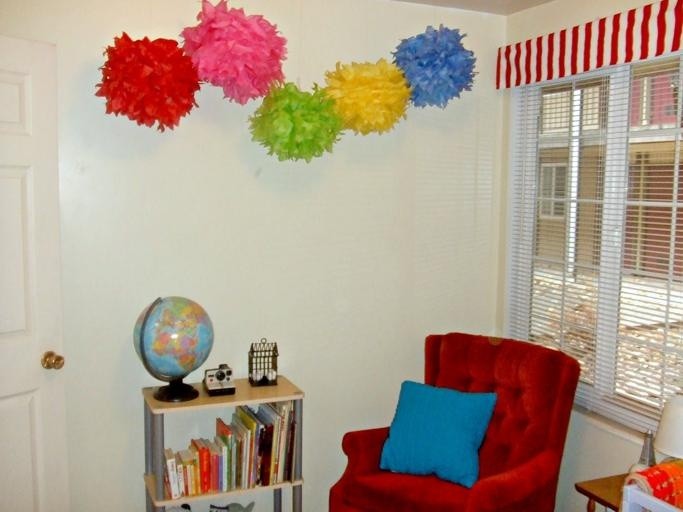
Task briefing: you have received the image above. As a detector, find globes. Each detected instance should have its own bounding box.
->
[134,296,214,403]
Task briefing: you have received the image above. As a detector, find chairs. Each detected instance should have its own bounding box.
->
[328,330,580,512]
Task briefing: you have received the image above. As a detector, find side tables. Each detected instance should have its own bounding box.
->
[574,472,630,512]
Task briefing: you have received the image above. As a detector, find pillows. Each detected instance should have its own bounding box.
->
[379,381,498,488]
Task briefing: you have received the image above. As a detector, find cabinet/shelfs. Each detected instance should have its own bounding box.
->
[142,375,305,512]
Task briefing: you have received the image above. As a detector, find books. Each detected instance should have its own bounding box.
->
[163,401,296,500]
